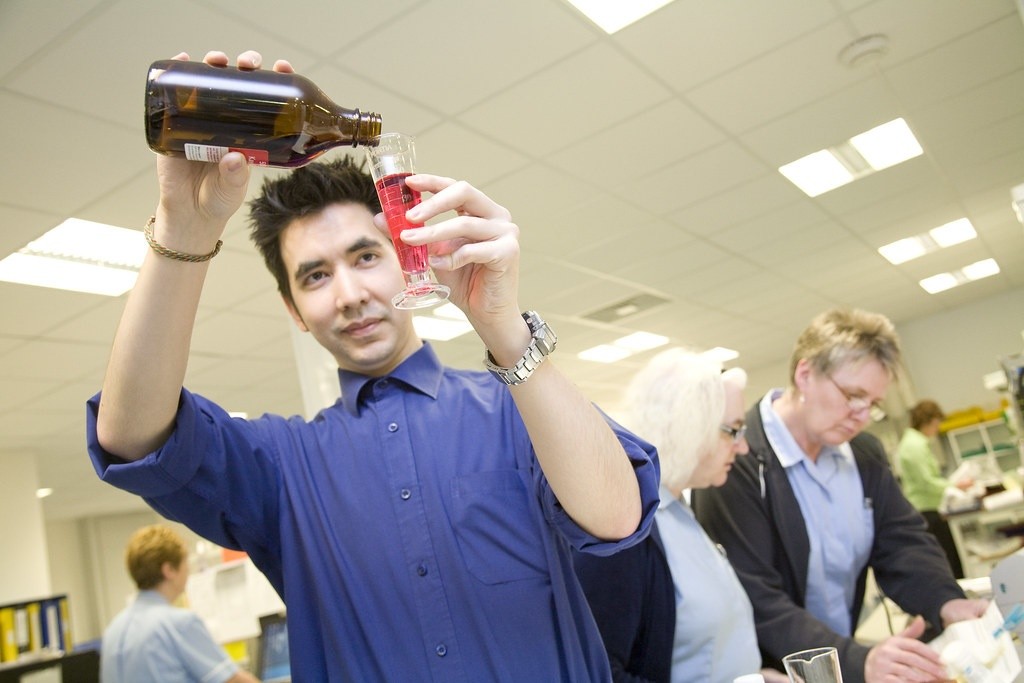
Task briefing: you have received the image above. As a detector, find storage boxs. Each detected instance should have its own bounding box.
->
[1,594,72,665]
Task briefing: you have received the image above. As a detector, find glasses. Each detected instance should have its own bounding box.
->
[826,372,888,423]
[722,425,749,441]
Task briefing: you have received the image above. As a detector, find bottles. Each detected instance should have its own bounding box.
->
[145,60,381,169]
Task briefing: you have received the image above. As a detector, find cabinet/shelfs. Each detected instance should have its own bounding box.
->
[938,411,1020,476]
[0,650,100,683]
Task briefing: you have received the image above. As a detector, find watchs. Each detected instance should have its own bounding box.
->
[480,307,558,386]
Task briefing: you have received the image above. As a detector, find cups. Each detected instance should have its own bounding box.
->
[781,647,843,683]
[363,132,451,309]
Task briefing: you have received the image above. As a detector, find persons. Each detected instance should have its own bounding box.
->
[894,399,976,578]
[613,346,787,683]
[98,522,266,683]
[691,305,992,683]
[83,48,661,682]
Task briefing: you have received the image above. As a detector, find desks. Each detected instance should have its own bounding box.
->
[940,500,1024,580]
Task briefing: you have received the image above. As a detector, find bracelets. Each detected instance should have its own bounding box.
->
[142,213,223,266]
